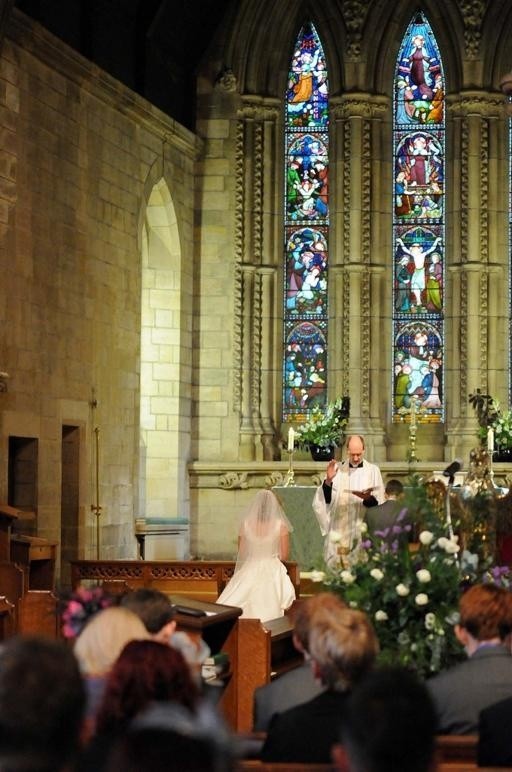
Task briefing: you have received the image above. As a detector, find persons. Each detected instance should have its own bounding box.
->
[311,434,386,572]
[1,634,236,772]
[252,594,439,772]
[214,488,298,624]
[362,478,418,550]
[430,584,512,767]
[77,589,175,712]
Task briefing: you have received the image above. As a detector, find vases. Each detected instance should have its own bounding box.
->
[495,447,512,461]
[309,444,334,461]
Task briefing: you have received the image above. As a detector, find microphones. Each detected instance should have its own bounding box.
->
[443,454,465,478]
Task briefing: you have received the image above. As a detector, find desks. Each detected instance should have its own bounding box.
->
[69,560,301,603]
[169,595,242,735]
[238,600,303,735]
[10,535,59,597]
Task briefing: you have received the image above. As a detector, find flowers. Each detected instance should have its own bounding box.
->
[306,504,512,680]
[294,397,348,453]
[476,397,512,450]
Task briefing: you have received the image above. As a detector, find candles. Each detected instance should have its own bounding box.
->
[288,427,294,451]
[488,428,493,451]
[411,395,418,427]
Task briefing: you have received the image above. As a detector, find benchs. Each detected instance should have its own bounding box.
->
[232,736,512,771]
